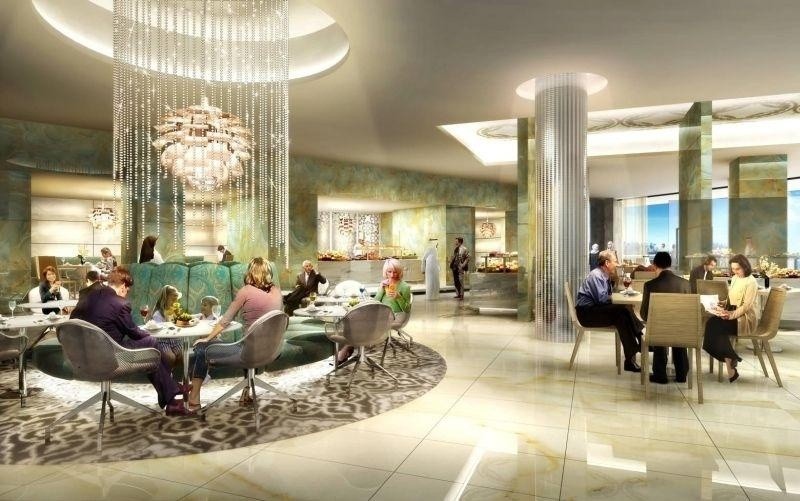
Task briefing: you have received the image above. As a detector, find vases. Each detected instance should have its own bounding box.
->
[176,320,187,325]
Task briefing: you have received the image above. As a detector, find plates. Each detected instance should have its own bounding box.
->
[145,325,164,332]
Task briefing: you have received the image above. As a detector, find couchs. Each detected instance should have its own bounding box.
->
[31,256,336,384]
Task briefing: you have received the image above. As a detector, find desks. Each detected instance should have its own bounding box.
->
[481,255,510,273]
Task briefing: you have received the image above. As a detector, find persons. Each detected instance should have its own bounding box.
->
[337,258,412,365]
[216,245,232,262]
[284,260,327,317]
[149,284,183,379]
[69,268,194,415]
[96,246,117,280]
[575,241,759,384]
[197,295,222,320]
[137,235,164,266]
[449,236,471,300]
[37,265,68,316]
[421,238,440,300]
[353,232,365,250]
[188,257,283,406]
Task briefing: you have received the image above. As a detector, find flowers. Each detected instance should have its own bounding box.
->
[167,302,192,321]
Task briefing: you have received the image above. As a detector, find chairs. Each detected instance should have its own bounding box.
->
[710,286,783,388]
[39,256,77,300]
[44,318,161,452]
[696,279,728,303]
[634,271,656,279]
[326,303,397,399]
[196,310,298,435]
[640,292,704,405]
[563,282,621,375]
[379,293,419,367]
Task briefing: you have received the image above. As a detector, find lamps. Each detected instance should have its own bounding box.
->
[113,1,291,270]
[88,200,118,230]
[480,209,496,239]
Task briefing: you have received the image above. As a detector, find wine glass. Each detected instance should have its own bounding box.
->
[60,256,66,265]
[9,300,17,320]
[58,300,65,316]
[139,304,149,323]
[309,292,317,311]
[622,277,633,296]
[210,304,222,324]
